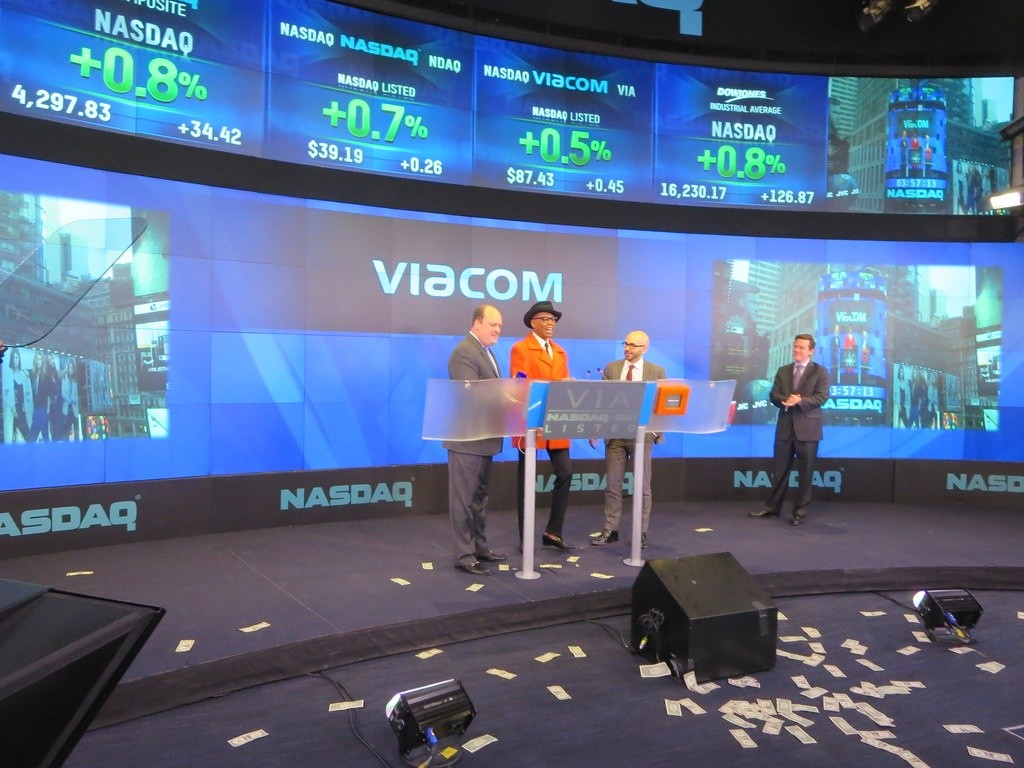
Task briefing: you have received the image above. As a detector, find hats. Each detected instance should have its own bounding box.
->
[524,301,561,328]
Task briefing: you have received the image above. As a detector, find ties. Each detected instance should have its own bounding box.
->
[626,365,635,381]
[794,365,804,390]
[545,343,548,352]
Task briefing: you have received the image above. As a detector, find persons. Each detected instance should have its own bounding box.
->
[0,345,82,445]
[896,366,944,432]
[504,301,581,555]
[902,130,999,217]
[448,304,509,579]
[831,325,871,383]
[587,331,667,550]
[746,334,829,526]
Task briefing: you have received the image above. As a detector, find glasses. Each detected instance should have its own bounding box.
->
[532,316,558,322]
[622,342,645,349]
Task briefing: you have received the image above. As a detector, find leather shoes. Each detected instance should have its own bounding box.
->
[791,516,802,525]
[747,509,779,519]
[474,552,507,561]
[456,563,493,575]
[640,535,647,548]
[542,533,576,549]
[591,530,618,545]
[520,540,523,553]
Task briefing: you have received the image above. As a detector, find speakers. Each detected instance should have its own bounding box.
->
[630,551,778,683]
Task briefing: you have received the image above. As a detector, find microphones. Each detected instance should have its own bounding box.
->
[597,368,608,380]
